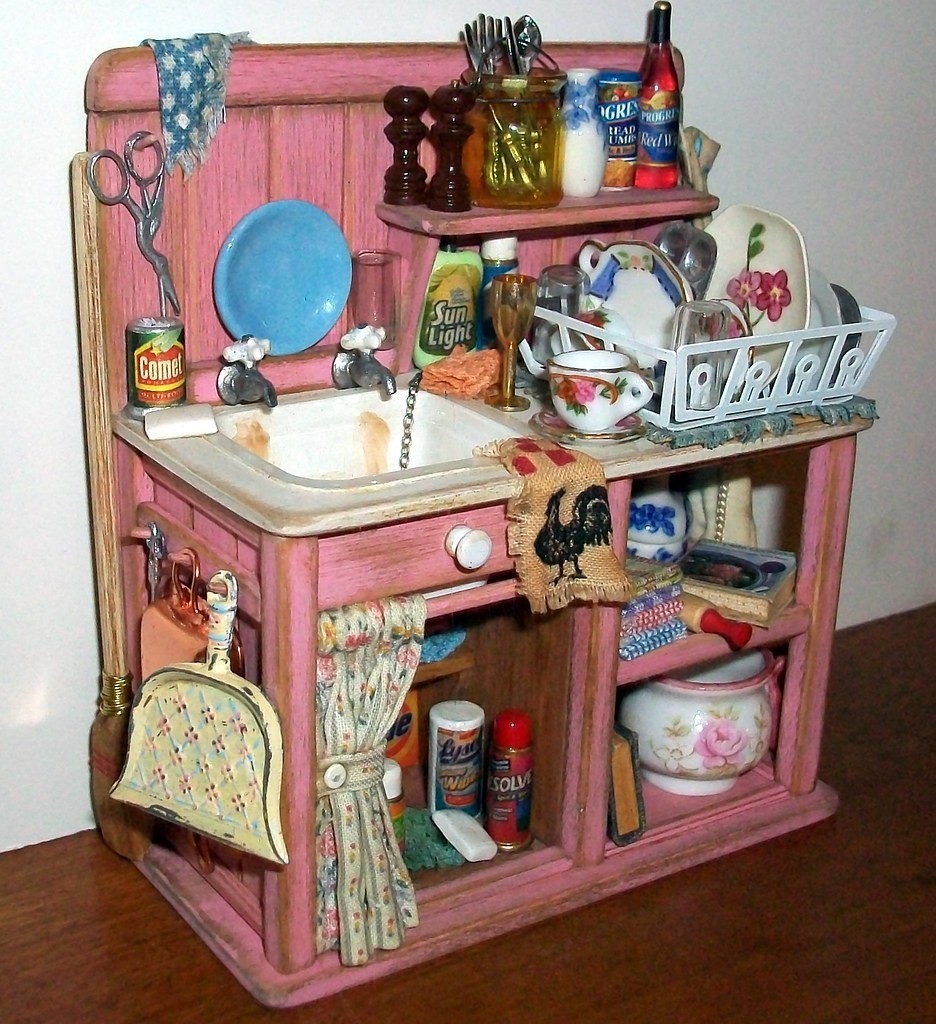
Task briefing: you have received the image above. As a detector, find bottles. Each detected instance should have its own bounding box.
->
[560,68,609,199]
[384,757,405,861]
[633,2,681,190]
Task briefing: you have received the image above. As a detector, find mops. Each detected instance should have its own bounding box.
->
[65,150,153,863]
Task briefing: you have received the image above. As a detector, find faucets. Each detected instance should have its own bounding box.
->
[330,323,397,395]
[215,334,277,408]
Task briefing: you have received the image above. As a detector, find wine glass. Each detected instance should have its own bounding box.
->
[484,275,534,409]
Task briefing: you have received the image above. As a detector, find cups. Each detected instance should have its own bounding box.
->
[545,350,654,433]
[672,301,729,410]
[464,71,561,210]
[534,266,591,363]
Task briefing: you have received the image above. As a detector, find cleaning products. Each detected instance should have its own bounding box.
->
[379,684,538,869]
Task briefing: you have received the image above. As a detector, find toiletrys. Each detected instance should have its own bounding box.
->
[414,234,520,371]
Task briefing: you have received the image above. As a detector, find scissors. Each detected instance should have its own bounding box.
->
[85,130,179,320]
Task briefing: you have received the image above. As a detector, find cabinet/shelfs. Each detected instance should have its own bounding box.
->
[115,416,876,1007]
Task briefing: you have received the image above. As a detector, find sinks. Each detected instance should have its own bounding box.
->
[209,385,530,492]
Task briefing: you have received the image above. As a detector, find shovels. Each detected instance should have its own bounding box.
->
[140,548,244,692]
[108,570,291,867]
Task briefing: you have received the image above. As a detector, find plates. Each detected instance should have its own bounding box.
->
[825,281,863,391]
[692,205,809,394]
[214,199,352,356]
[576,238,703,394]
[788,268,843,394]
[535,409,650,445]
